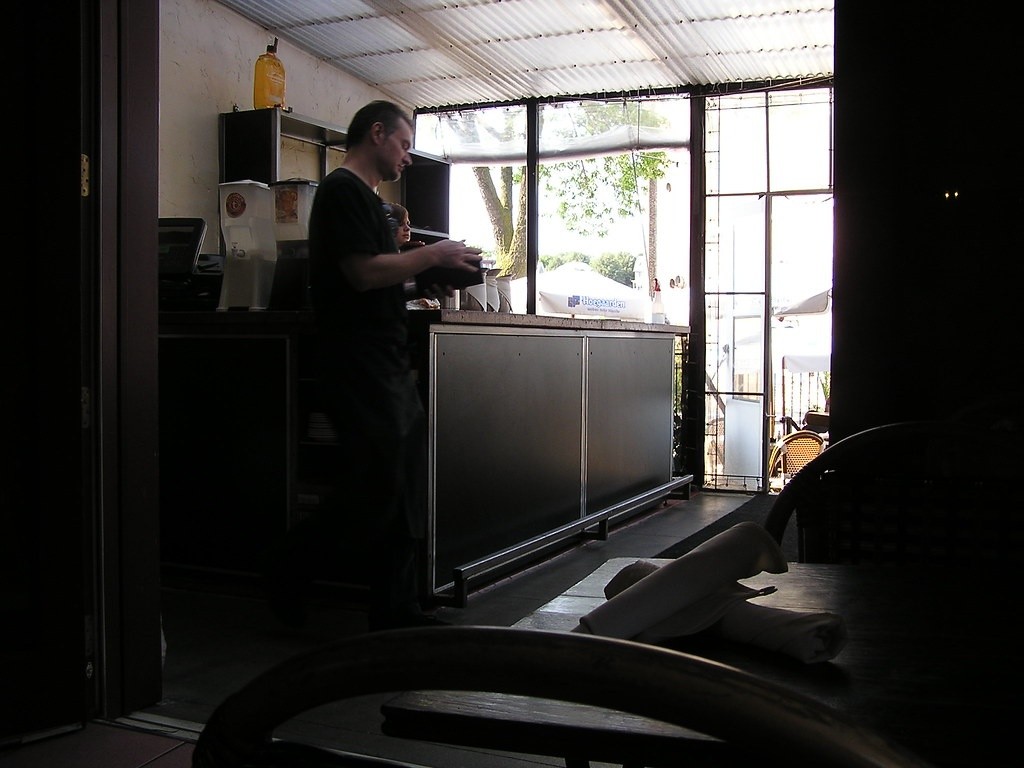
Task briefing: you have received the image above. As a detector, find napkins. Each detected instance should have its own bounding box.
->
[603,558,848,663]
[569,521,789,645]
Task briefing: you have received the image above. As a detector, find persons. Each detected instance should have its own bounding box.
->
[227,99,483,631]
[384,202,411,244]
[669,275,681,288]
[653,277,661,297]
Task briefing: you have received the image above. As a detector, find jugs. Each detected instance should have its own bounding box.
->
[438,267,512,313]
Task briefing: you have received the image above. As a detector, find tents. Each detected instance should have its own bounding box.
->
[510,260,652,324]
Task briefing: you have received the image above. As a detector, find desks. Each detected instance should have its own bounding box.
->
[380,553,1024,768]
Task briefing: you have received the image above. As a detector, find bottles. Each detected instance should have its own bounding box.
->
[254,37,286,110]
[652,288,665,324]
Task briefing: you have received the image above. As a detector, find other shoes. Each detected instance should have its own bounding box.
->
[369,614,452,632]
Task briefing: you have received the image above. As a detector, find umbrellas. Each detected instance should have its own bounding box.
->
[774,287,833,320]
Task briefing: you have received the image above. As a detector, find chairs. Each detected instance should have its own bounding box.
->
[766,430,827,494]
[705,417,761,488]
[763,418,1024,564]
[192,628,929,768]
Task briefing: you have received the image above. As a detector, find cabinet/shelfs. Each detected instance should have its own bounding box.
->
[218,106,452,253]
